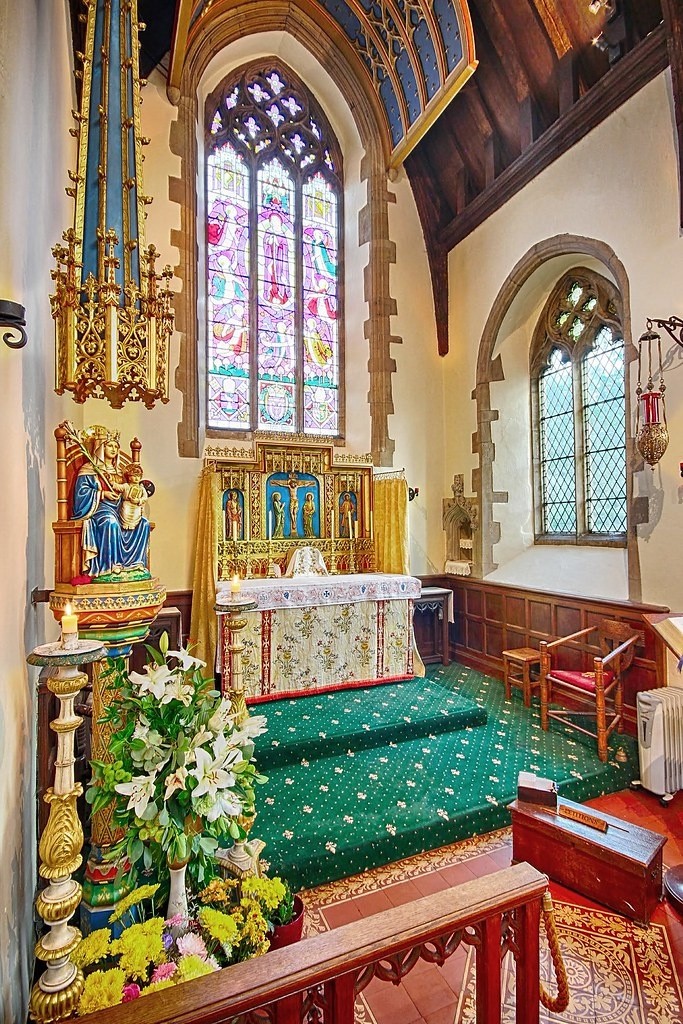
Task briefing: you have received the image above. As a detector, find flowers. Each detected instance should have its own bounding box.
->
[58,420,115,493]
[67,631,296,1016]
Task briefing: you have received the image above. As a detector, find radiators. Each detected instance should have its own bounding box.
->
[629,686,683,808]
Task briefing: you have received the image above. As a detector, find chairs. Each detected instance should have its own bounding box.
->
[540,619,640,761]
[52,420,155,581]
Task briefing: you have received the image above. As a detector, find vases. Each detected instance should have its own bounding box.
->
[167,864,188,928]
[264,893,305,952]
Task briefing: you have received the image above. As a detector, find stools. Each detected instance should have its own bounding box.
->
[502,647,551,707]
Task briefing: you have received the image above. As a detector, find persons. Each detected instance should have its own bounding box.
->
[64,425,149,584]
[272,480,316,539]
[225,490,244,540]
[339,492,356,538]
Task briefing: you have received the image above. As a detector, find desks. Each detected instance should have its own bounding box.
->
[413,587,454,666]
[506,796,669,931]
[85,607,183,682]
[217,573,426,704]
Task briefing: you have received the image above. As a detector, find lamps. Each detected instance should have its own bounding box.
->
[589,0,612,16]
[591,31,609,54]
[635,316,683,474]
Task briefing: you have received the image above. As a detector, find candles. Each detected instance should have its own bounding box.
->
[222,510,225,542]
[331,510,334,539]
[61,604,78,633]
[371,511,373,542]
[233,521,237,541]
[231,575,240,592]
[354,521,358,538]
[348,511,352,539]
[269,510,272,541]
[247,508,249,542]
